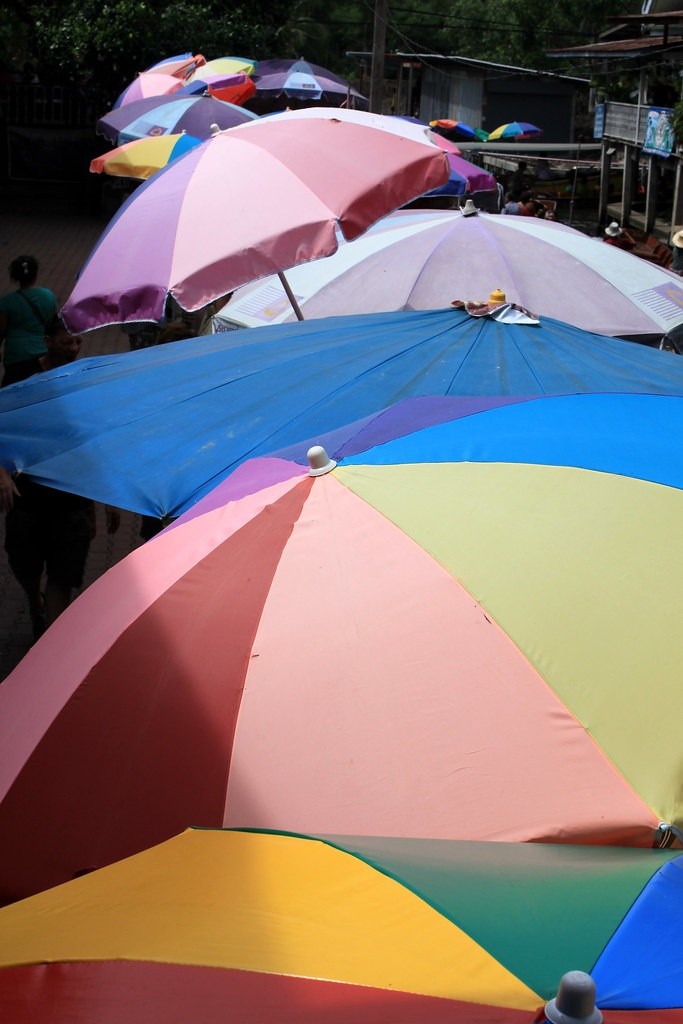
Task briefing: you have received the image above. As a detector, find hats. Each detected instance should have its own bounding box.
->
[605,222,622,236]
[672,230,683,248]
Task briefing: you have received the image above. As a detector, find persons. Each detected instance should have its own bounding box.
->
[0,314,120,641]
[136,318,209,541]
[1,256,62,385]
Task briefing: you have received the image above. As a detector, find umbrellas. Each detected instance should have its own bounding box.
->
[0,52,683,1024]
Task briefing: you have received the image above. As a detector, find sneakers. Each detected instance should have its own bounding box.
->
[29,592,48,638]
